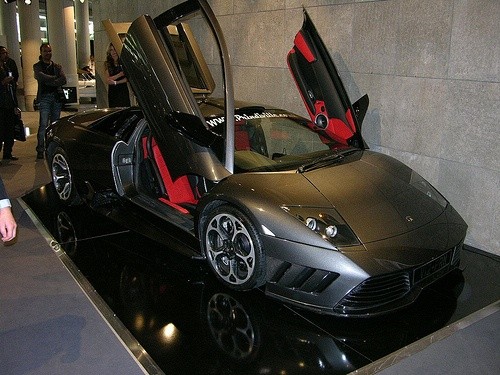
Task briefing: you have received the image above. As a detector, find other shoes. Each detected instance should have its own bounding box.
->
[36,151,44,160]
[2,156,19,161]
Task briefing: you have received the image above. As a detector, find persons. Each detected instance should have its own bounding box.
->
[0,176,18,242]
[104,43,131,108]
[33,43,67,158]
[88,55,95,76]
[0,46,19,161]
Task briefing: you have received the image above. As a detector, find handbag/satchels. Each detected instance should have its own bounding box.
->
[14,108,26,143]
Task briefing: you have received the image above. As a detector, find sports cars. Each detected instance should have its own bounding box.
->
[44,1,470,321]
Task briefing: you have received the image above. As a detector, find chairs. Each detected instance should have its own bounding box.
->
[147,120,250,205]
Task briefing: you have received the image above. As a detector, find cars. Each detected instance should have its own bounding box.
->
[78,68,96,98]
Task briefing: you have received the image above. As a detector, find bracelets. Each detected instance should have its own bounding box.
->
[114,81,116,86]
